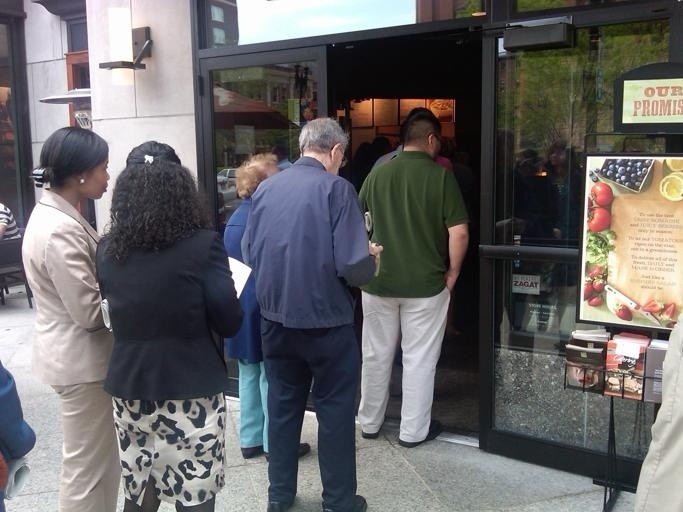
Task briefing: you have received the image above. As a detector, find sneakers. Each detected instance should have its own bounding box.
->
[322,495,367,511]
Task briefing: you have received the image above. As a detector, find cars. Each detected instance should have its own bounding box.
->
[217,168,239,202]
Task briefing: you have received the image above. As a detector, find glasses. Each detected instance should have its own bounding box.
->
[428,131,441,144]
[337,147,348,168]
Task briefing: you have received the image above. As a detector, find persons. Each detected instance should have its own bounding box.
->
[633,311,683,512]
[497,128,585,352]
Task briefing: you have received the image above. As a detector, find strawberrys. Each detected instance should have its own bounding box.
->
[658,304,677,326]
[613,303,632,321]
[641,297,666,312]
[584,268,606,307]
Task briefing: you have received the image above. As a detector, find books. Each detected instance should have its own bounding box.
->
[566,330,669,403]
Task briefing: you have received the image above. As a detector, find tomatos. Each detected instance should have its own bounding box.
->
[587,183,613,233]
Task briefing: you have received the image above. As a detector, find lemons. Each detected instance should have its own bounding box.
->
[659,159,683,201]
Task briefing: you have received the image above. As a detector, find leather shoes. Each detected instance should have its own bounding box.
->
[240,444,263,458]
[266,443,310,462]
[398,420,441,448]
[362,415,387,439]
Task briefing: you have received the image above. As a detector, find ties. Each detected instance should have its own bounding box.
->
[267,494,296,512]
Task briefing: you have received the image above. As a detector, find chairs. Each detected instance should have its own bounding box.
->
[0,238,33,309]
